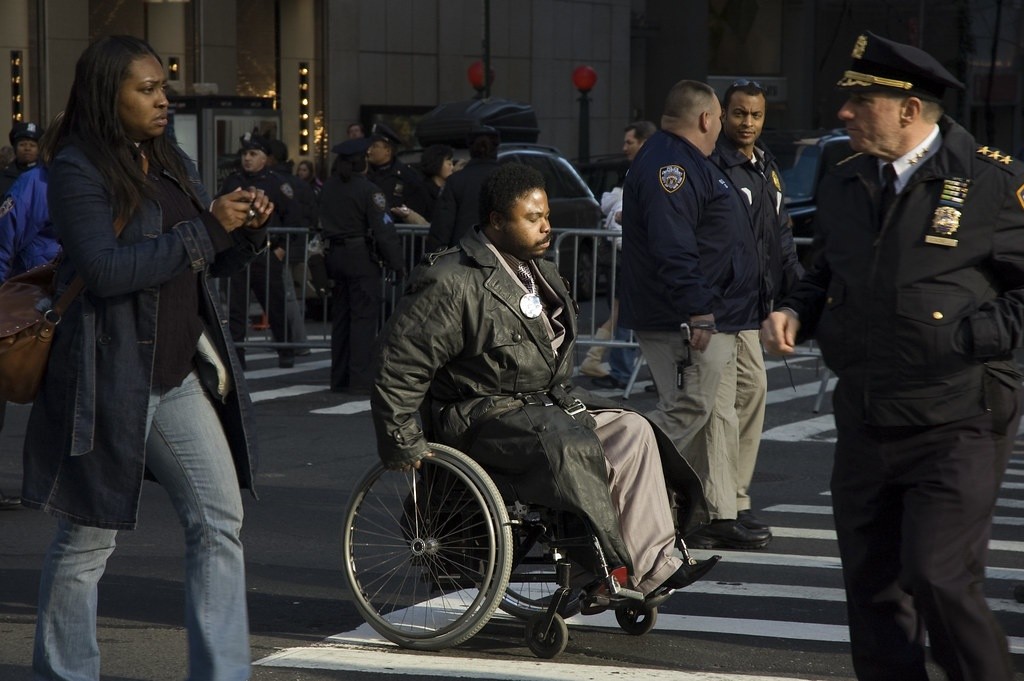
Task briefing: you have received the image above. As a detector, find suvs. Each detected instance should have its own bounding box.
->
[395,95,633,303]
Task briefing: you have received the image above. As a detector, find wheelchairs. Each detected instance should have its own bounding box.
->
[337,442,699,659]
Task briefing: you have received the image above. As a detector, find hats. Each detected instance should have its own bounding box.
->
[9,122,45,146]
[331,138,372,159]
[240,134,272,157]
[834,29,967,101]
[369,123,403,145]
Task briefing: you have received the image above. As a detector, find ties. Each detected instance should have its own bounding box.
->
[876,164,898,234]
[517,262,556,340]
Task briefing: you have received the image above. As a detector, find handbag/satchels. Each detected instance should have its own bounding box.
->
[0,261,63,405]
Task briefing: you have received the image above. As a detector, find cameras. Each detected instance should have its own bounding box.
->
[237,198,256,222]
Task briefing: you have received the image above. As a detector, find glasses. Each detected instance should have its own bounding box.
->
[732,79,768,92]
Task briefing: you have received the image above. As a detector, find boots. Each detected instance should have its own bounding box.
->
[577,328,615,378]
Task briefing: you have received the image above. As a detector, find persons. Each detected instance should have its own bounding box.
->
[0,111,62,508]
[707,79,798,532]
[222,123,499,396]
[32,36,275,681]
[762,31,1024,681]
[371,165,722,599]
[579,121,654,389]
[617,79,771,551]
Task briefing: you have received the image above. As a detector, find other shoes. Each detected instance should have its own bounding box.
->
[347,384,373,396]
[331,383,348,394]
[297,350,312,356]
[645,385,657,392]
[590,376,627,389]
[279,351,293,368]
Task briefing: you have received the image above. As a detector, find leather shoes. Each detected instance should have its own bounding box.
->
[581,567,628,616]
[740,510,769,530]
[646,555,719,600]
[686,519,773,549]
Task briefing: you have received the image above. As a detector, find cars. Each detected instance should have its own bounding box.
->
[759,127,864,267]
